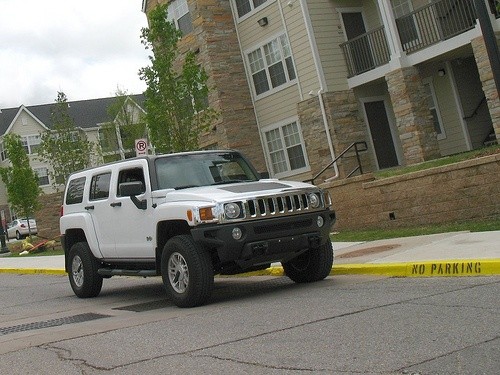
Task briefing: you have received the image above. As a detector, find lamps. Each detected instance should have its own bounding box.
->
[437,68,445,78]
[257,16,268,27]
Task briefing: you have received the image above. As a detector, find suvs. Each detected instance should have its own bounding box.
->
[59,148,337,309]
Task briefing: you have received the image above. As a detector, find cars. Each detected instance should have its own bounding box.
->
[4,217,37,240]
